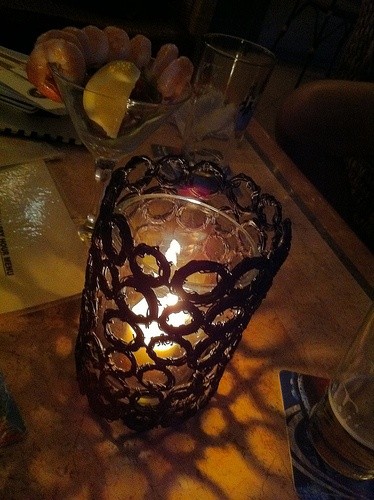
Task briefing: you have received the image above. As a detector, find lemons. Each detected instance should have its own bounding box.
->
[82,59,141,139]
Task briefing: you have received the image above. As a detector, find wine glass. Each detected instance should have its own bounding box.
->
[46,58,194,232]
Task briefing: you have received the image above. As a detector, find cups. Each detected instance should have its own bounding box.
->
[312,302,374,481]
[75,155,292,430]
[193,33,278,143]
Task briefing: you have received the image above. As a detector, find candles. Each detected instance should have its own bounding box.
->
[120,295,185,404]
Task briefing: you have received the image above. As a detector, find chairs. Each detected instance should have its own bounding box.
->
[274,81,373,251]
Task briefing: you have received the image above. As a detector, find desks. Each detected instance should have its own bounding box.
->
[0,120,372,499]
